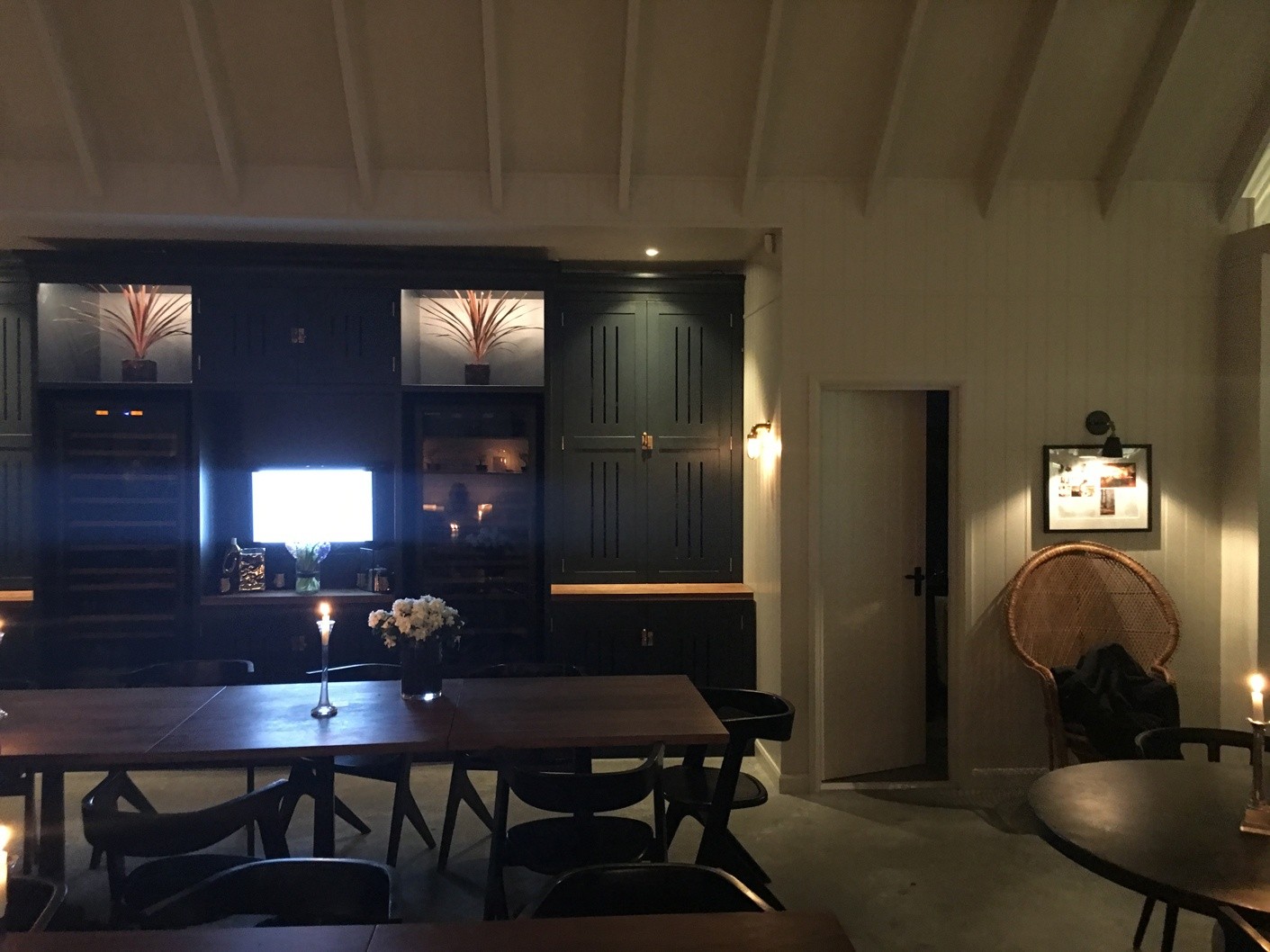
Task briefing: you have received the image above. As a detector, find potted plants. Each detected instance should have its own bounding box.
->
[52,284,194,383]
[417,290,544,385]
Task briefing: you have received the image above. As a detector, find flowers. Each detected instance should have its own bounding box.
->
[367,593,466,694]
[289,538,331,591]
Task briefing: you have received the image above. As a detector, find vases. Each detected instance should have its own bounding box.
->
[296,570,320,595]
[400,636,443,699]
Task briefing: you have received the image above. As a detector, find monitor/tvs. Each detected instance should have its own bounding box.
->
[250,466,376,555]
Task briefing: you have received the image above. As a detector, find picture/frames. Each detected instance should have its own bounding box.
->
[1044,444,1152,533]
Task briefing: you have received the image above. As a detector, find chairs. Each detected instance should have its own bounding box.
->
[1002,535,1181,772]
[1134,727,1270,952]
[0,660,795,933]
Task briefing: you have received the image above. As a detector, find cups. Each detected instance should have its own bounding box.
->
[371,567,389,593]
[237,548,268,593]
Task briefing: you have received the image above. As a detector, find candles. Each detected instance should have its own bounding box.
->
[319,601,333,644]
[1246,671,1269,723]
[0,825,12,920]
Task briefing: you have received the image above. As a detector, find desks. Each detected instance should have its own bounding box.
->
[147,674,731,860]
[1027,755,1270,952]
[0,685,224,882]
[0,908,854,952]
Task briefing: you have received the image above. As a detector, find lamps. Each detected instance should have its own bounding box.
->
[746,419,771,459]
[1085,410,1123,458]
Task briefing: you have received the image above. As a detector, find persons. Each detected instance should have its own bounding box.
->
[422,482,530,577]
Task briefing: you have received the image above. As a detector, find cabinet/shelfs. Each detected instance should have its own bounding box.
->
[57,403,190,643]
[548,270,754,689]
[412,401,533,660]
